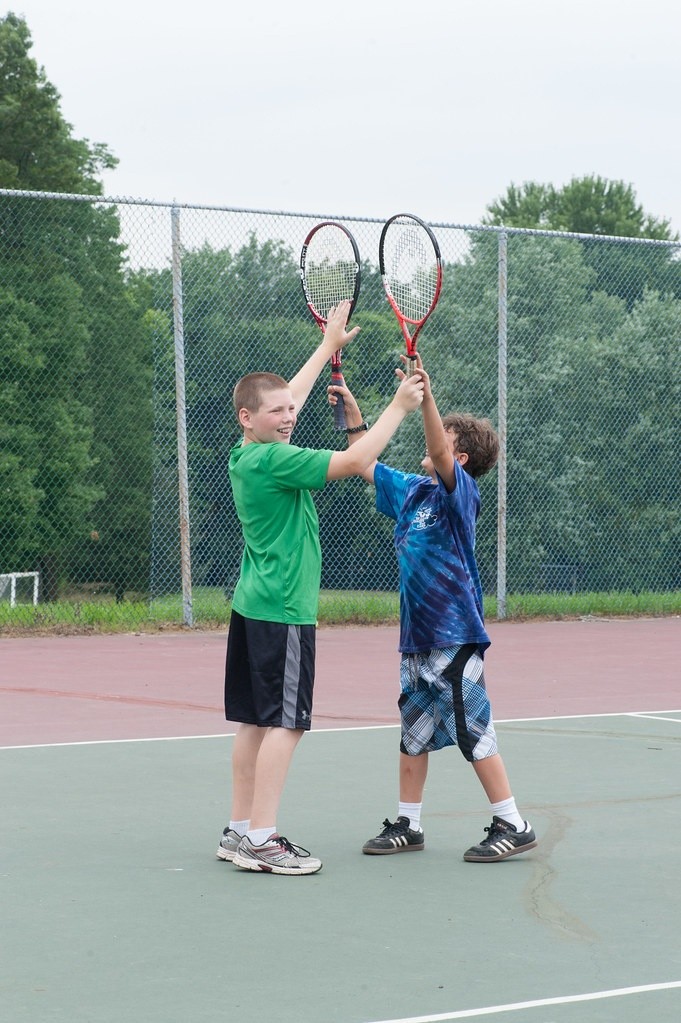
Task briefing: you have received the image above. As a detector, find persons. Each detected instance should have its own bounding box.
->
[330,352,538,862]
[216,300,425,876]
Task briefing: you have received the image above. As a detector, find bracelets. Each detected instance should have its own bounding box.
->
[345,422,368,433]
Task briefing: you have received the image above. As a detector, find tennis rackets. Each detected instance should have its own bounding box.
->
[299,221,364,432]
[376,213,444,376]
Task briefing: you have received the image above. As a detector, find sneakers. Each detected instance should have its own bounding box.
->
[362,815,424,855]
[231,832,323,876]
[463,815,536,862]
[216,827,243,861]
